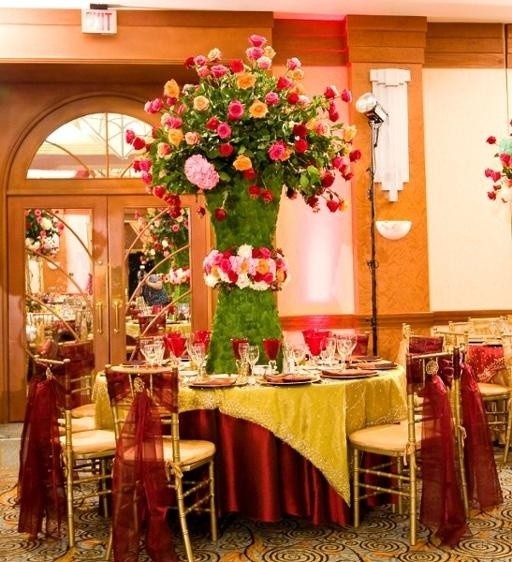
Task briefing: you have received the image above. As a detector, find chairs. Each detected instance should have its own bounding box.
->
[24,296,94,341]
[17,341,117,548]
[103,365,216,562]
[404,316,511,462]
[126,303,191,352]
[348,346,468,545]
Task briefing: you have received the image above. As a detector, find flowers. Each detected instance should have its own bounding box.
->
[125,33,362,376]
[483,119,512,205]
[134,207,191,304]
[23,208,65,260]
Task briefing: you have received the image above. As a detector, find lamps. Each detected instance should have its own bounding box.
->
[354,90,389,355]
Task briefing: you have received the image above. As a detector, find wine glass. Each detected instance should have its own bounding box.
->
[138,332,305,379]
[318,331,357,370]
[126,294,187,326]
[29,290,96,342]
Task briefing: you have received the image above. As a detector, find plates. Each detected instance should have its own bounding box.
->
[318,362,400,379]
[351,354,385,362]
[187,377,319,388]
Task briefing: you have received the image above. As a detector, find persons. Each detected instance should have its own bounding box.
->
[142,261,170,305]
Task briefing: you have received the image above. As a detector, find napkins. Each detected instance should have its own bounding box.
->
[352,363,398,367]
[265,374,312,382]
[322,369,376,375]
